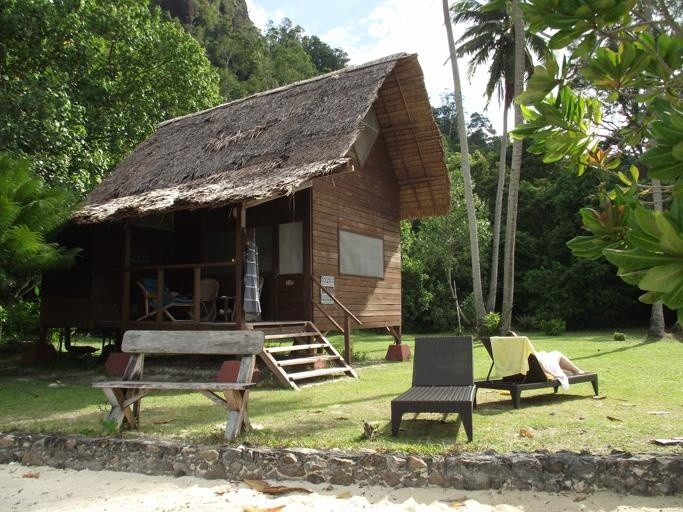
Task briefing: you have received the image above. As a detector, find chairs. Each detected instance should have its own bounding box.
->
[391,335,477,442]
[136,276,265,322]
[473,335,598,410]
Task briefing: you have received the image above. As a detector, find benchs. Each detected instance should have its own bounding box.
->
[90,329,264,443]
